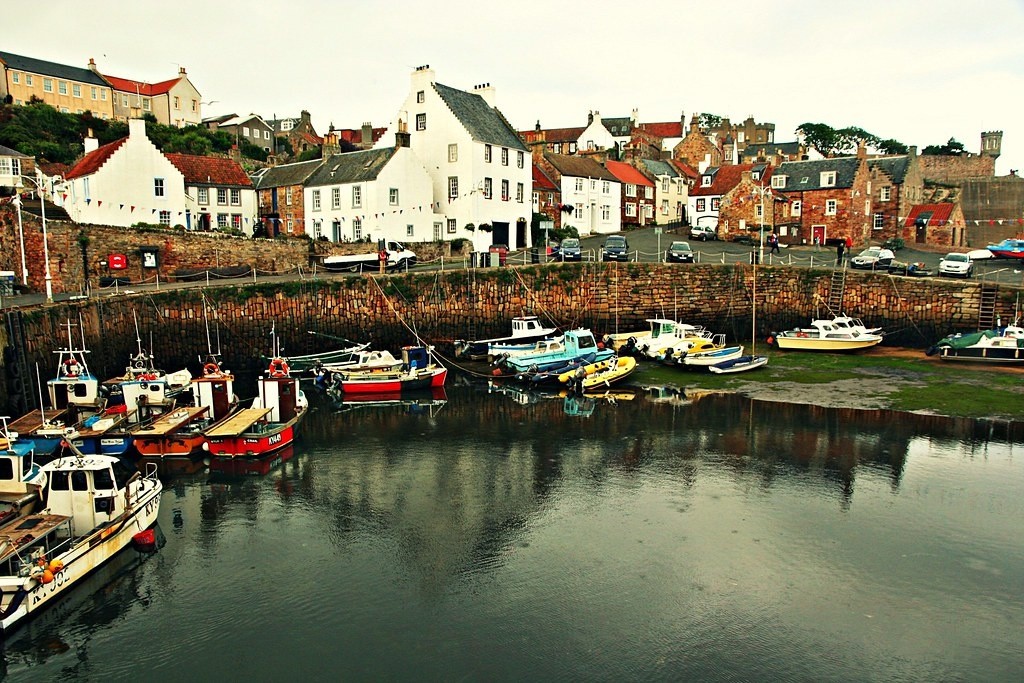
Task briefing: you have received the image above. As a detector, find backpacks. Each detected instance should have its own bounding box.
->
[773,236,779,245]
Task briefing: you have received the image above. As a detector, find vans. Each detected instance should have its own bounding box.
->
[601,234,630,262]
[556,238,584,261]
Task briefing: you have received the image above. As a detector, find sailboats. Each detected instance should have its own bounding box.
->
[708,245,770,374]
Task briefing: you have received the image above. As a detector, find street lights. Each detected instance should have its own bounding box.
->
[12,174,62,303]
[747,180,772,264]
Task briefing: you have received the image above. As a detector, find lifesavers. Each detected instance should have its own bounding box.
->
[269,359,289,378]
[795,332,808,338]
[62,359,82,377]
[203,363,219,376]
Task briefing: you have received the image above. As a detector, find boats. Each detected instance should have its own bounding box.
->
[986,238,1024,260]
[926,291,1024,365]
[0,437,48,527]
[0,434,164,633]
[0,291,745,490]
[903,261,934,276]
[771,294,884,351]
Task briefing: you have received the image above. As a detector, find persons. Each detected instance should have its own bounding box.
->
[837,243,844,265]
[379,248,390,274]
[390,248,402,273]
[814,234,822,253]
[770,232,780,254]
[845,236,852,257]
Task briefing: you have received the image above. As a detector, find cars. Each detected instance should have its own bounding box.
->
[937,252,975,278]
[850,246,896,270]
[667,241,695,264]
[688,225,715,242]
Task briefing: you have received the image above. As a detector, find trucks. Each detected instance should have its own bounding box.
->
[324,240,417,273]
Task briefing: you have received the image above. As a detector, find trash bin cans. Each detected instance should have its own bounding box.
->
[531,248,539,263]
[481,252,489,268]
[489,244,507,265]
[469,251,477,268]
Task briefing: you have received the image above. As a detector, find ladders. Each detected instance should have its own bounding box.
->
[826,259,848,320]
[977,269,1000,332]
[9,312,36,410]
[305,280,315,355]
[97,300,112,376]
[467,270,475,341]
